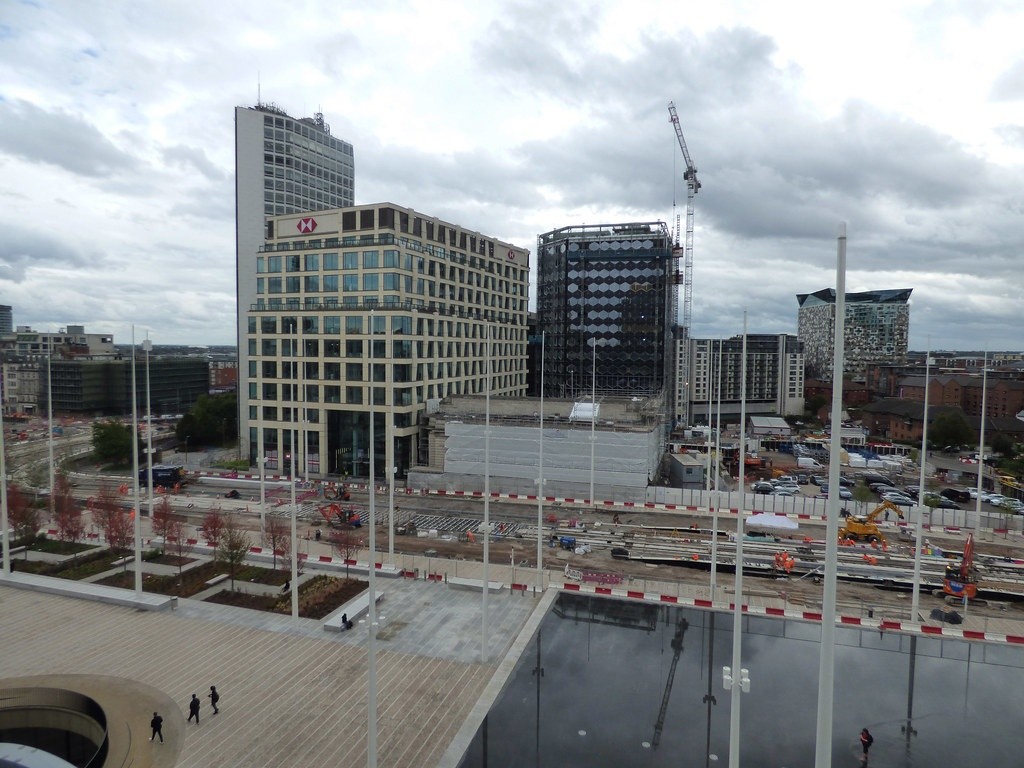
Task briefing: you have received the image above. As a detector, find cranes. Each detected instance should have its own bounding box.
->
[668,100,701,338]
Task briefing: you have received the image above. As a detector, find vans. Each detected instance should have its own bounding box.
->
[797,457,825,470]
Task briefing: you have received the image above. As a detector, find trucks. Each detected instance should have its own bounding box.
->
[136,463,188,489]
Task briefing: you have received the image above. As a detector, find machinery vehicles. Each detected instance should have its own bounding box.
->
[838,499,906,543]
[931,533,989,609]
[323,485,350,501]
[319,502,361,530]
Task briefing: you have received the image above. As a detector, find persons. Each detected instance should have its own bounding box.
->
[885,510,889,521]
[149,712,164,744]
[208,686,219,715]
[775,549,794,573]
[131,507,135,524]
[154,482,181,494]
[925,537,930,548]
[119,483,128,496]
[838,536,887,565]
[187,694,200,724]
[467,529,476,543]
[282,580,290,593]
[858,728,869,762]
[947,562,955,580]
[342,613,351,630]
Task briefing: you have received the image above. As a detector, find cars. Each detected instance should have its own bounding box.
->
[748,475,1023,515]
